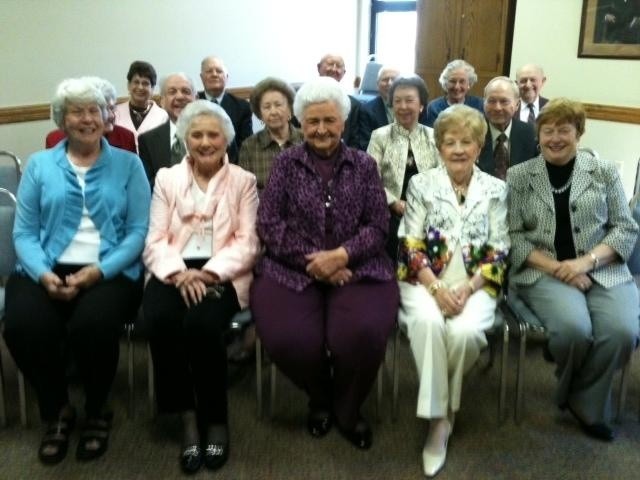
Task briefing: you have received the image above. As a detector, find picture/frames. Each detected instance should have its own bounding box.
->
[577,0,640,61]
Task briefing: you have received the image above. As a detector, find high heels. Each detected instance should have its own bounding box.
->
[421,419,453,478]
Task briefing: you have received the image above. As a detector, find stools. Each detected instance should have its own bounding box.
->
[264,356,387,422]
[17,320,138,428]
[388,315,514,430]
[146,305,264,424]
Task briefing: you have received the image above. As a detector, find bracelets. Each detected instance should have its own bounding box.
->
[429,281,449,296]
[585,251,598,271]
[465,281,476,294]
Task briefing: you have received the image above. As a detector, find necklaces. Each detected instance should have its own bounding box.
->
[552,172,573,193]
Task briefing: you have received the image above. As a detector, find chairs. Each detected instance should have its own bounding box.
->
[503,147,639,425]
[0,186,26,430]
[354,53,386,102]
[2,147,23,195]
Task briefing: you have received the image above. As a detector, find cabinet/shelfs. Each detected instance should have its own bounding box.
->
[409,0,515,100]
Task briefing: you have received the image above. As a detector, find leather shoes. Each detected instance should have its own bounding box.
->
[573,409,615,442]
[74,407,114,462]
[334,405,374,450]
[307,405,334,439]
[178,429,203,476]
[37,406,78,466]
[203,421,230,471]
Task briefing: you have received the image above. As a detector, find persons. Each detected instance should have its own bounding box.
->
[140,99,262,474]
[365,74,442,215]
[111,54,255,193]
[506,96,639,442]
[3,77,151,464]
[397,104,512,477]
[318,53,399,152]
[250,75,400,450]
[428,60,551,177]
[235,76,306,193]
